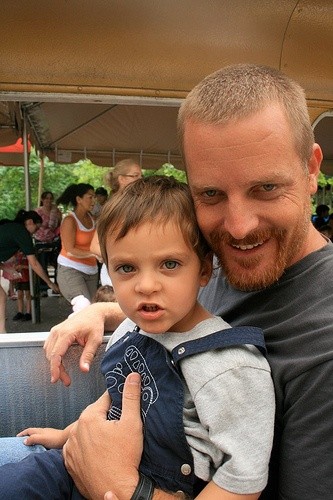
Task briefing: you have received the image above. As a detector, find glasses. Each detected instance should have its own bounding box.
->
[123,174,145,181]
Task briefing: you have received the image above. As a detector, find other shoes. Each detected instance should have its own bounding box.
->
[11,293,17,299]
[13,312,24,320]
[21,313,32,321]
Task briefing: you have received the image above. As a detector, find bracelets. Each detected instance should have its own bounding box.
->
[129,472,155,500]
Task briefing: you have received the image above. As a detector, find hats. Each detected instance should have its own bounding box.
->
[95,187,107,195]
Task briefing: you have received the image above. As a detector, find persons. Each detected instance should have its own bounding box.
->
[0,66,333,500]
[0,160,333,335]
[16,175,275,500]
